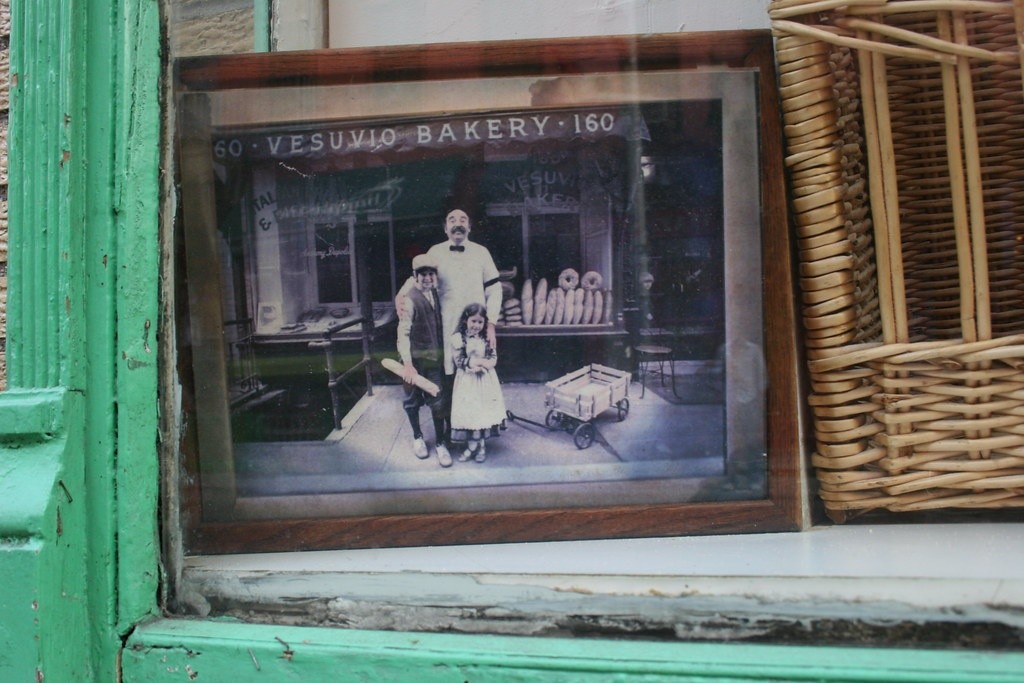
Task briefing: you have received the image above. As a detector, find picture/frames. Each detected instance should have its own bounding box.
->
[169,28,802,558]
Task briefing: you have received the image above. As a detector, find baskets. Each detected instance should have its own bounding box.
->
[768,0,1024,524]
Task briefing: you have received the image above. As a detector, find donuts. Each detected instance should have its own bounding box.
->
[558,268,579,291]
[582,271,602,291]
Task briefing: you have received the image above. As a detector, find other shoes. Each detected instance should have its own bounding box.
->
[414,438,428,458]
[435,446,452,467]
[475,448,486,462]
[458,448,476,461]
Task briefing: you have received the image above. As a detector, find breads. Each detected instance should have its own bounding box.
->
[381,358,439,398]
[497,278,603,324]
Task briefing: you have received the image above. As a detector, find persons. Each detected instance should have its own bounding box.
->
[395,210,508,466]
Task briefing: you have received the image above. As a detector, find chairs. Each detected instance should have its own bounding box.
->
[634,301,681,399]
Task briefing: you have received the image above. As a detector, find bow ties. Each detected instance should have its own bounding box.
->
[450,245,465,252]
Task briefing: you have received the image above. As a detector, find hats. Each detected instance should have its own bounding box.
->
[412,254,439,270]
